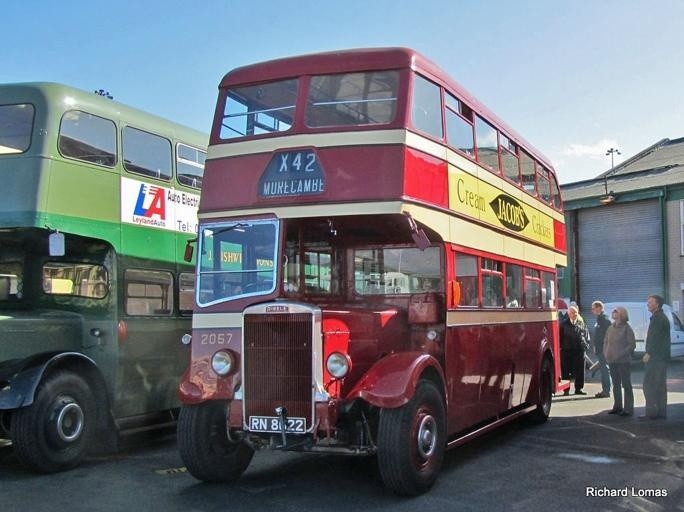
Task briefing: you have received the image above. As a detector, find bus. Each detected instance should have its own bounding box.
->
[0,79,421,475]
[174,46,572,498]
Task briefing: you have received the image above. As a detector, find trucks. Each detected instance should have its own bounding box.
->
[602,301,684,366]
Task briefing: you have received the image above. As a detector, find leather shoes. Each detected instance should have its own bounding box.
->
[609,410,648,419]
[564,390,586,395]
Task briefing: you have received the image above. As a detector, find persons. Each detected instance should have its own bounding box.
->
[592,300,612,399]
[602,306,636,418]
[636,296,670,420]
[559,298,591,396]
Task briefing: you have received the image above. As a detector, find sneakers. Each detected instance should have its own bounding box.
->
[596,392,610,397]
